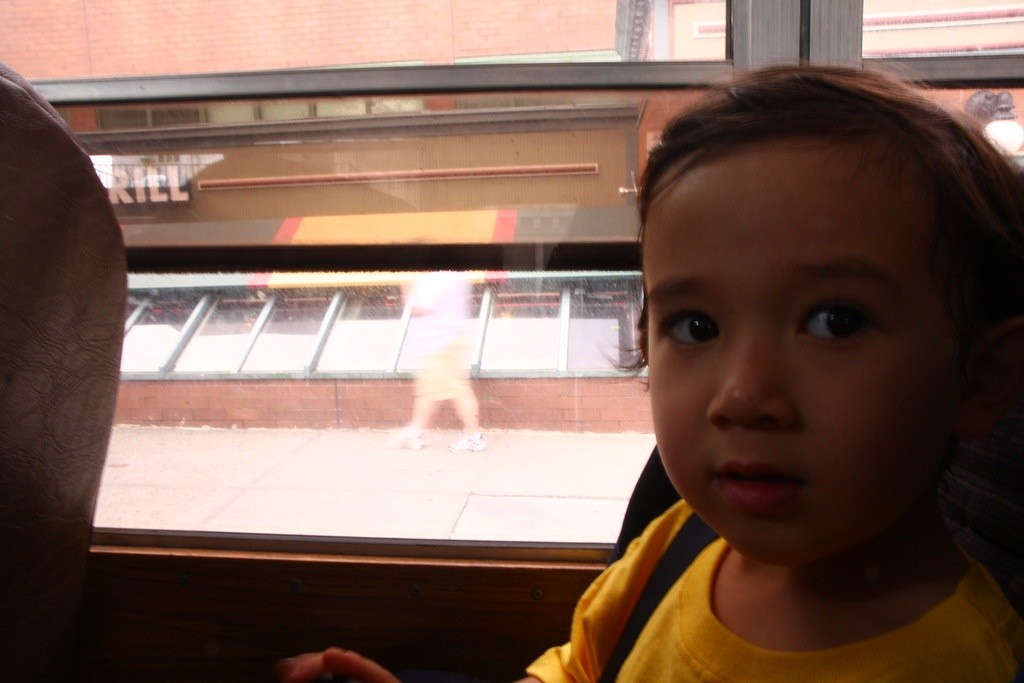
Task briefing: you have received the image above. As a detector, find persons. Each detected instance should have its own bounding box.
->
[391,280,488,451]
[276,62,1023,682]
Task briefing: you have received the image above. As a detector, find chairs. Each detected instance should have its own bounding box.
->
[0,61,128,683]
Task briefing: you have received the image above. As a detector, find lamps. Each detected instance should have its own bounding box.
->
[964,91,1023,157]
